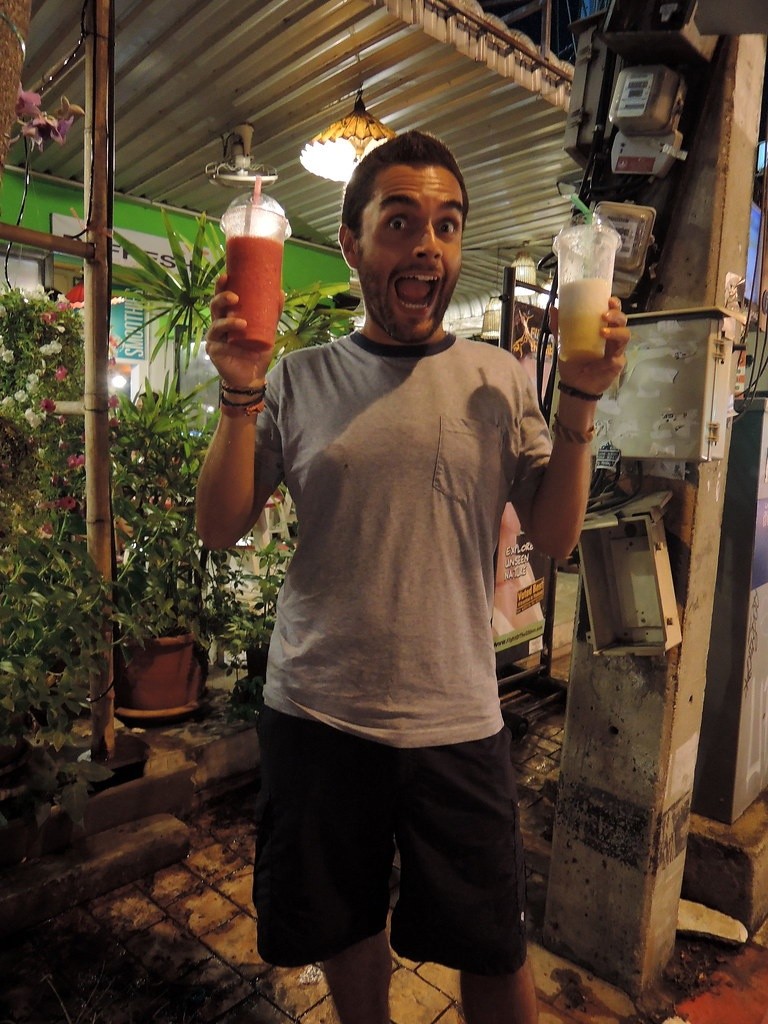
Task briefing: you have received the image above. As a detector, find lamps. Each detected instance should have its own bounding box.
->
[480,296,504,338]
[510,250,537,297]
[298,90,400,183]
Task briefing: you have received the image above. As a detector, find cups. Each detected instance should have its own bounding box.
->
[219,188,292,349]
[551,210,623,361]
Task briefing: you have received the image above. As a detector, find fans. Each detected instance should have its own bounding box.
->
[203,126,278,190]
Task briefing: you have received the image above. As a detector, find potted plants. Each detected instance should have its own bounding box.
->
[110,208,360,719]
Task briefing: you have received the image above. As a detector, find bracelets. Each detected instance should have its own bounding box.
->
[219,382,267,414]
[552,414,595,443]
[557,382,604,401]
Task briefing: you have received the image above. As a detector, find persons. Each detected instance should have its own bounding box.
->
[139,391,159,405]
[490,499,543,637]
[194,128,630,1024]
